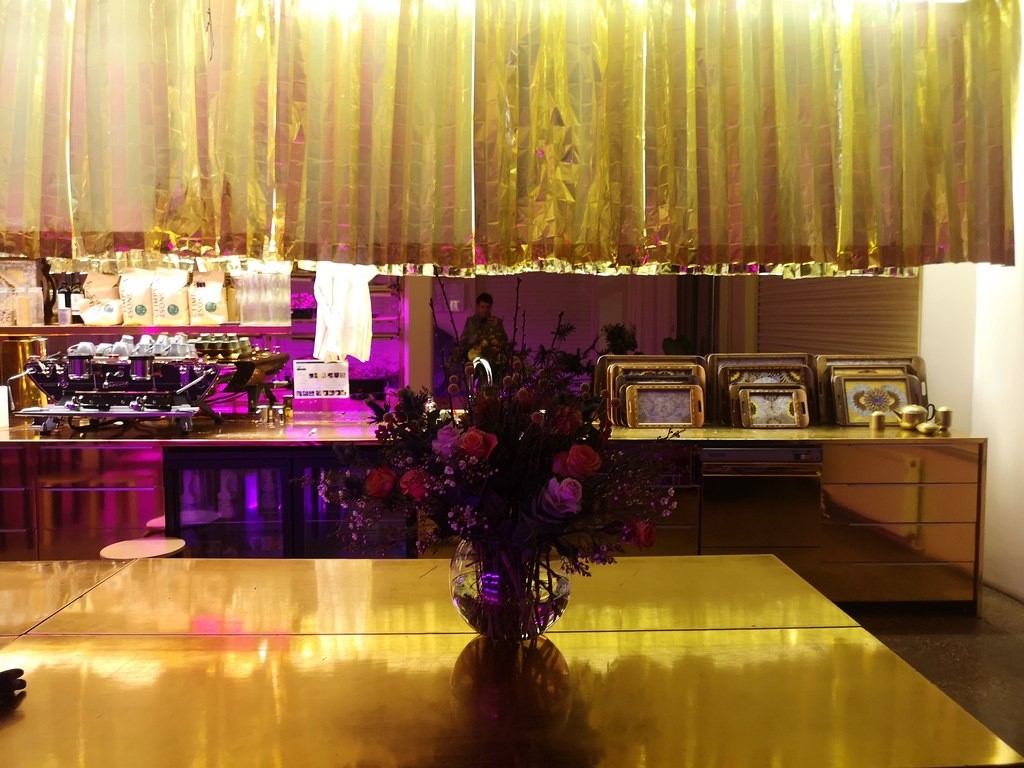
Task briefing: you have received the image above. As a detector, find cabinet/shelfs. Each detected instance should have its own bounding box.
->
[1,406,988,618]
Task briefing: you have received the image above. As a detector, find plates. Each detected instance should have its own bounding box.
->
[100,539,185,560]
[145,508,221,529]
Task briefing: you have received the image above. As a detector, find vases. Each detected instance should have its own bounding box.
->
[446,537,579,747]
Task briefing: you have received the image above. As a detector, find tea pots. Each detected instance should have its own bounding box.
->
[892,402,935,429]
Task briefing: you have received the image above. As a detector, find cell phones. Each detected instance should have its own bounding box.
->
[595,354,929,429]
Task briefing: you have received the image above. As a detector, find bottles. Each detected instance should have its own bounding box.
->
[871,409,885,430]
[935,406,953,431]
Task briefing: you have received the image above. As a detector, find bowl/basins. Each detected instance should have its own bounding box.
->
[917,425,939,434]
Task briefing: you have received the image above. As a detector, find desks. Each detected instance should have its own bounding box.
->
[1,553,1022,767]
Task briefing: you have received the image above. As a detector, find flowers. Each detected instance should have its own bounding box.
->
[361,263,685,703]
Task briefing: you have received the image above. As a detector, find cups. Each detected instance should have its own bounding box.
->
[71,331,252,357]
[271,405,286,424]
[255,405,270,422]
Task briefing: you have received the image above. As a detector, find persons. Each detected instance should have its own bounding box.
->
[459,292,509,349]
